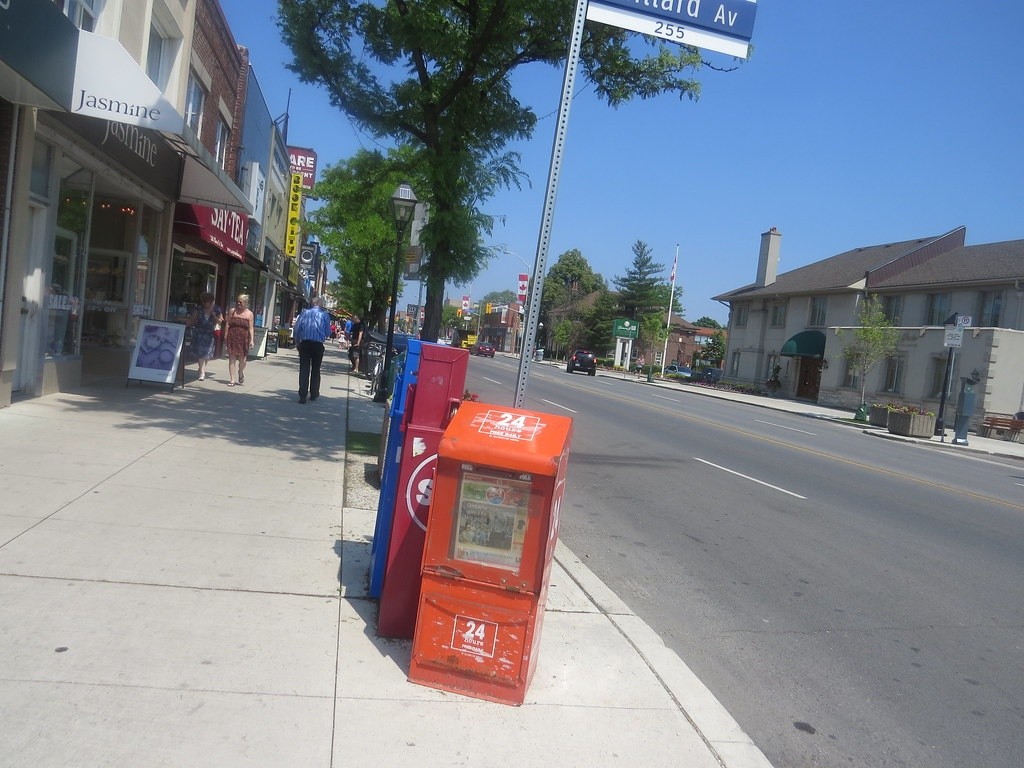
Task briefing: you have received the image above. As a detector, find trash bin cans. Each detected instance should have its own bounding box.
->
[365,342,388,380]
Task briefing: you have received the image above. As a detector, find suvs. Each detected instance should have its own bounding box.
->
[566,348,599,377]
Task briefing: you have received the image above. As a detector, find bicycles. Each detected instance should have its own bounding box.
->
[365,342,389,396]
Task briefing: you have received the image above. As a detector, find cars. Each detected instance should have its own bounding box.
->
[471,342,496,359]
[664,364,697,380]
[389,332,421,353]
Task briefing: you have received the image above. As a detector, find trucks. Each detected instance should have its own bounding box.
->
[451,330,478,354]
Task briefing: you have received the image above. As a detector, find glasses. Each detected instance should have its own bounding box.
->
[235,301,245,303]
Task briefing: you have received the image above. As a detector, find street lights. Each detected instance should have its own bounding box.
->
[373,183,419,403]
[503,250,532,358]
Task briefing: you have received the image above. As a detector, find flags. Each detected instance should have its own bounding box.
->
[670,254,676,281]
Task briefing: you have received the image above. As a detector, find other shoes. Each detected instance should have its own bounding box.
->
[311,394,319,400]
[230,382,235,386]
[239,376,244,383]
[198,376,205,381]
[299,397,306,403]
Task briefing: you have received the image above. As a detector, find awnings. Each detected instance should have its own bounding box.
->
[778,330,827,358]
[71,27,255,215]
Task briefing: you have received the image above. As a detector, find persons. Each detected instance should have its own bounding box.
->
[633,354,645,379]
[347,314,366,375]
[223,294,254,387]
[292,297,333,404]
[255,310,263,327]
[185,292,224,381]
[329,317,352,351]
